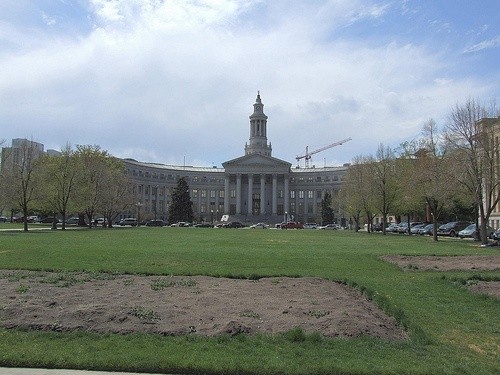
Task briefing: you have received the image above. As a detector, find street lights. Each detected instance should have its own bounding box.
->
[154,184,160,227]
[135,201,143,227]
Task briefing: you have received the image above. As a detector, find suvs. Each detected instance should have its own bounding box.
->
[66,217,80,224]
[120,218,137,226]
[39,217,59,224]
[91,217,109,225]
[399,222,422,234]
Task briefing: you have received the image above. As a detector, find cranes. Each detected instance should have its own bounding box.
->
[296,137,352,168]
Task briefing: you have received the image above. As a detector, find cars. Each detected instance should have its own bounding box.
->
[0,215,39,223]
[417,223,442,236]
[145,220,193,227]
[373,222,402,233]
[492,227,500,240]
[436,221,471,237]
[249,223,269,229]
[216,221,242,229]
[411,225,425,234]
[458,223,496,239]
[195,222,214,228]
[274,222,342,230]
